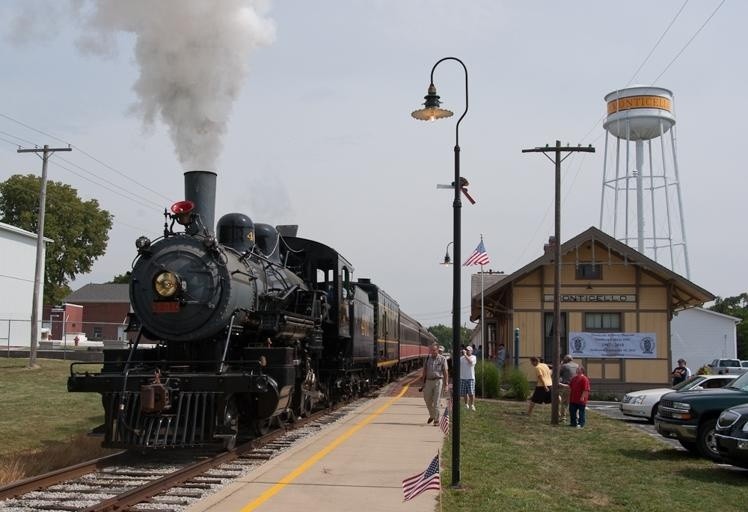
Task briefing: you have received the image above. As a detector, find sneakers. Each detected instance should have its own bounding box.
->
[464,404,476,412]
[427,417,440,426]
[576,424,585,431]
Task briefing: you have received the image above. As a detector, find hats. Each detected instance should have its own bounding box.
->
[465,345,474,351]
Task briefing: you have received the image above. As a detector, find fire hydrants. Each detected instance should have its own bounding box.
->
[73,335,79,346]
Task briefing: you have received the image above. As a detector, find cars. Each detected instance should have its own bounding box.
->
[618,358,748,472]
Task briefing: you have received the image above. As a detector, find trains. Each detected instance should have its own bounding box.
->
[66,170,439,466]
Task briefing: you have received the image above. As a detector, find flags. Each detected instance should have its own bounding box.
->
[441,407,450,437]
[403,454,441,502]
[462,240,490,266]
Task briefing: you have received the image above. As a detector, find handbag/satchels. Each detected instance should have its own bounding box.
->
[422,354,429,384]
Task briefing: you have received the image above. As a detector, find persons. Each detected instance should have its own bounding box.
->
[415,336,453,426]
[521,351,590,429]
[460,340,508,410]
[671,357,709,386]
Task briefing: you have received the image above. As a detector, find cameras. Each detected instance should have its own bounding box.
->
[460,350,464,356]
[418,385,425,393]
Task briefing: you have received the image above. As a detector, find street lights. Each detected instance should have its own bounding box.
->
[414,57,472,486]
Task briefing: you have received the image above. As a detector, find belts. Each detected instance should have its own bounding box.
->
[427,376,445,380]
